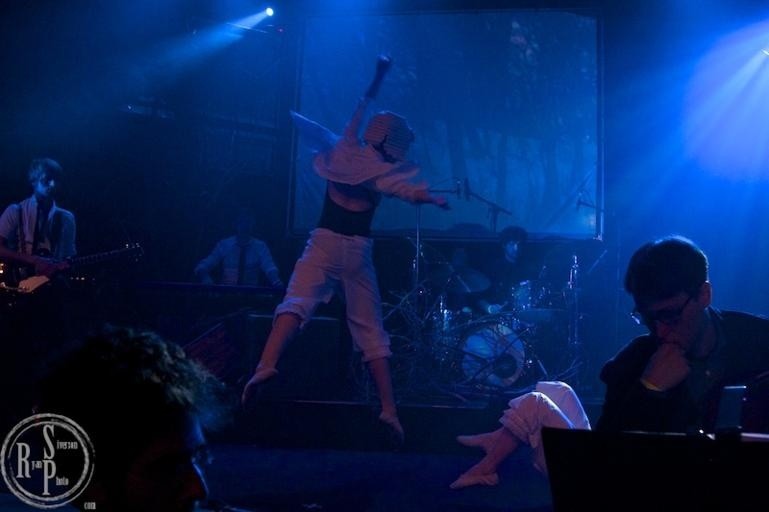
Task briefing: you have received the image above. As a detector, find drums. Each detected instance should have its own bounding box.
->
[512,280,571,325]
[459,321,538,387]
[433,309,471,362]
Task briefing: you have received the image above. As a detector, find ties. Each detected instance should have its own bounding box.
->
[238,247,246,283]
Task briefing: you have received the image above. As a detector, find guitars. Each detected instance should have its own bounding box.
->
[0,242,144,306]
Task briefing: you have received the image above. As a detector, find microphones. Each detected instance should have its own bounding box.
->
[575,192,584,211]
[455,179,464,201]
[462,174,472,203]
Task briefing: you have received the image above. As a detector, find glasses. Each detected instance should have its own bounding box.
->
[628,292,699,329]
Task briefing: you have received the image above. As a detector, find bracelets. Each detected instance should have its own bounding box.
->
[486,305,493,314]
[271,279,282,286]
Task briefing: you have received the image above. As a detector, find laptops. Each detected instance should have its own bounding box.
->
[538,425,769,510]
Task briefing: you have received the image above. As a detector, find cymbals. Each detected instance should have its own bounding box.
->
[408,236,446,260]
[526,236,577,244]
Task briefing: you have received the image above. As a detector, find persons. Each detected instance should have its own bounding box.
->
[240,52,453,446]
[190,203,288,286]
[446,379,593,492]
[465,224,543,316]
[594,236,769,437]
[30,321,230,512]
[0,155,78,284]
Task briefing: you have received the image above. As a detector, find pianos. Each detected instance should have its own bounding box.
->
[136,281,285,303]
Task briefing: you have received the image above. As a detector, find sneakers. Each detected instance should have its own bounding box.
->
[240,366,279,406]
[379,410,406,446]
[450,469,500,490]
[456,432,493,454]
